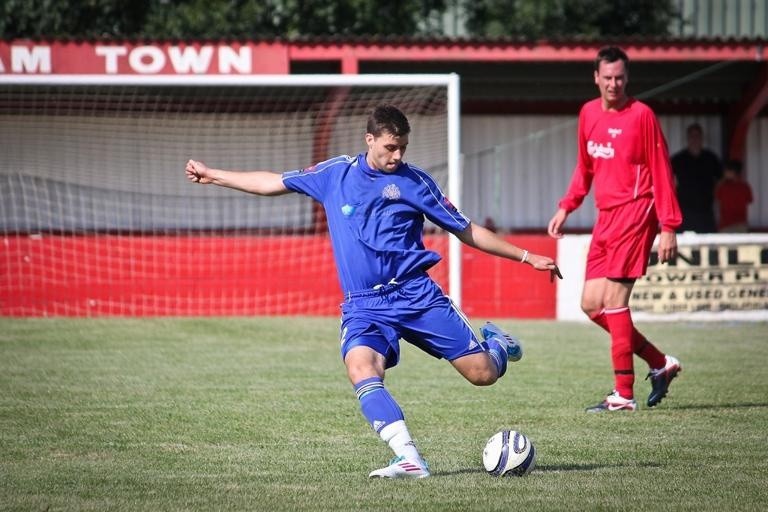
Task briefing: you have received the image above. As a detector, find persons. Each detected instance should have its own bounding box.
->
[668,121,722,232]
[547,45,684,410]
[711,157,753,232]
[184,101,565,482]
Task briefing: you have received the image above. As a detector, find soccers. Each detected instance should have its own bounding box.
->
[482,428,537,477]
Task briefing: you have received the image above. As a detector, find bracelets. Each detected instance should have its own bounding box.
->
[520,248,528,264]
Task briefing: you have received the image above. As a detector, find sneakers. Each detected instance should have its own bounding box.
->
[585,392,637,413]
[479,321,523,362]
[647,354,682,407]
[368,455,431,480]
